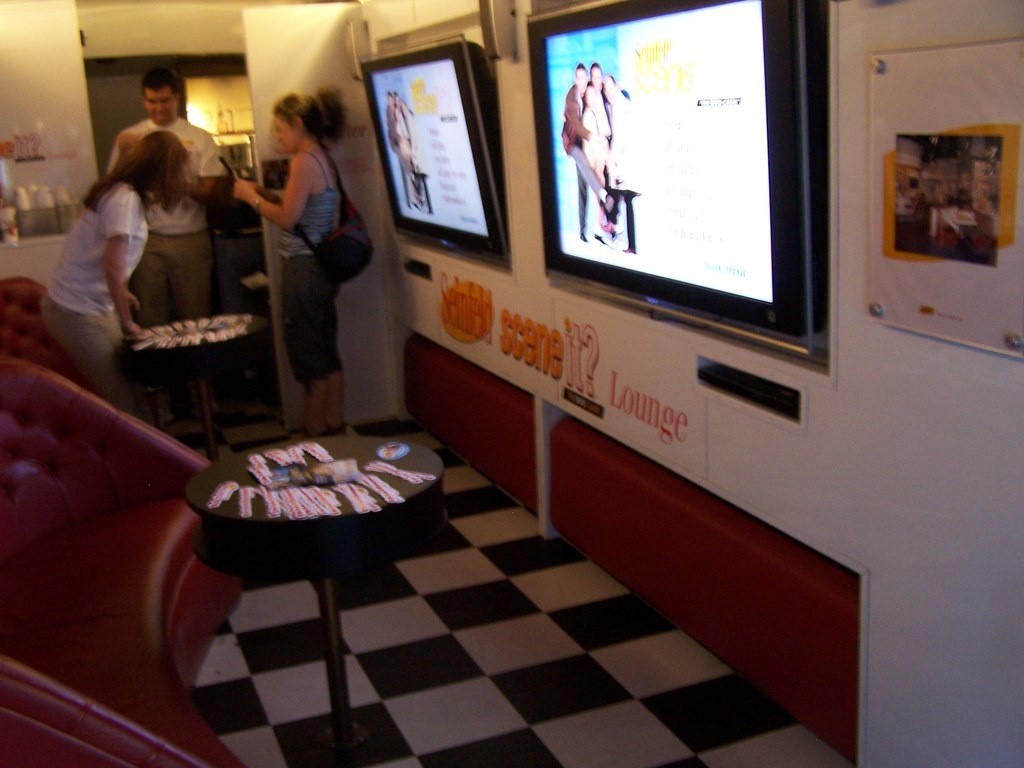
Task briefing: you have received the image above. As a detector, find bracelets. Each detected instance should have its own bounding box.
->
[254,199,259,215]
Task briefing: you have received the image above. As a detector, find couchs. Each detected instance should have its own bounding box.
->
[0,355,246,768]
[0,277,90,386]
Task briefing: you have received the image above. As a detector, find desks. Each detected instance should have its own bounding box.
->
[186,437,447,750]
[122,312,270,462]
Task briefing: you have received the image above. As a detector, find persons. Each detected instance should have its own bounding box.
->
[385,89,435,216]
[41,130,192,420]
[107,58,231,402]
[561,63,644,255]
[232,91,349,440]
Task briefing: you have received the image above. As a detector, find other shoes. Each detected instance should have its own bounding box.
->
[580,235,588,242]
[602,223,615,234]
[624,249,638,253]
[595,234,606,245]
[604,195,613,212]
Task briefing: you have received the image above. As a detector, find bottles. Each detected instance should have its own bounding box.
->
[14,183,74,235]
[218,112,229,134]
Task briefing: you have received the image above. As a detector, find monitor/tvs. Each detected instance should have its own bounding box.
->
[359,39,512,270]
[526,0,818,358]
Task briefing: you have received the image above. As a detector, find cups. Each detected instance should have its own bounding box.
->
[0,207,18,242]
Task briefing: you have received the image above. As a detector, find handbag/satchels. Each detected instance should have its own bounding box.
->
[313,188,372,283]
